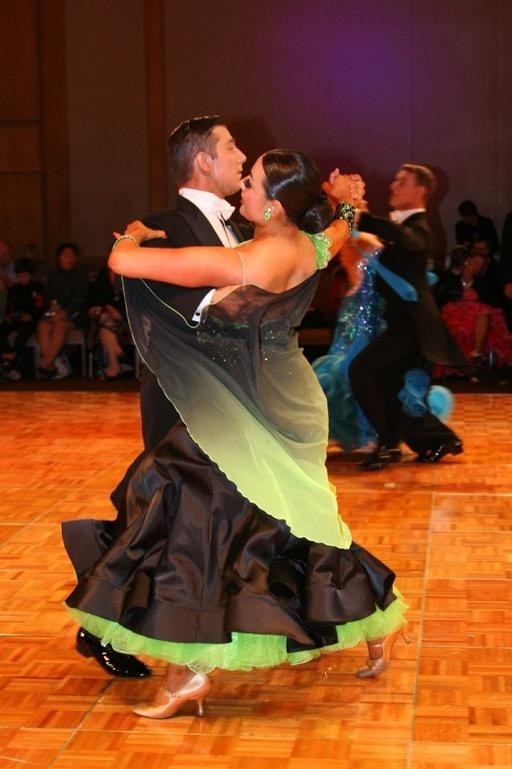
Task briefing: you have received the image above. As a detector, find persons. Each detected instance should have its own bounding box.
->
[309,178,384,450]
[60,114,366,681]
[2,197,509,393]
[63,149,414,721]
[345,163,467,473]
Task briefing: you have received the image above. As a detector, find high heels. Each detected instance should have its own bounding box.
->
[132,670,212,719]
[355,621,411,679]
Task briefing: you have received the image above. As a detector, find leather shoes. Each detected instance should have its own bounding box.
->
[414,438,463,463]
[77,627,152,679]
[356,446,401,470]
